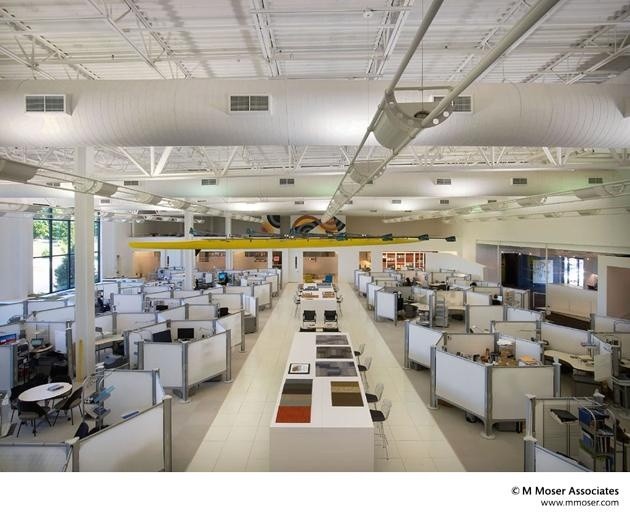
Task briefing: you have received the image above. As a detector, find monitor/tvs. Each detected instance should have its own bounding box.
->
[156,305,168,311]
[0,334,16,345]
[153,330,172,342]
[178,328,194,342]
[219,308,228,317]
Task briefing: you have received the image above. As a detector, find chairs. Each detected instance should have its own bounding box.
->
[293,284,343,312]
[355,342,393,462]
[8,374,84,439]
[299,310,338,332]
